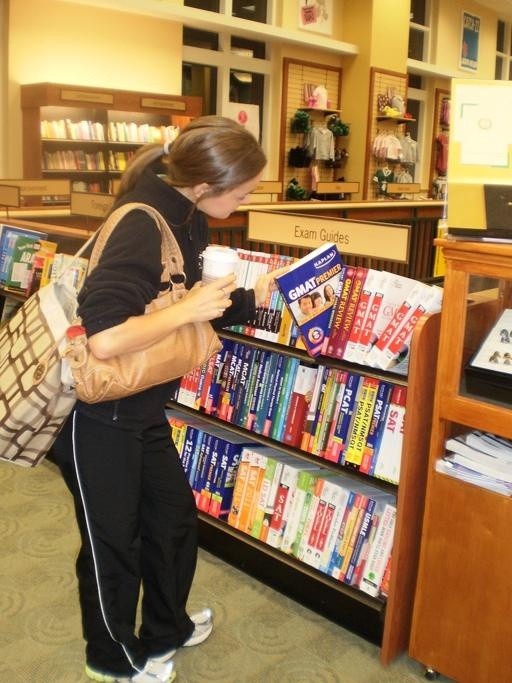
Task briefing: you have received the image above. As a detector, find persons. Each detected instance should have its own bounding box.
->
[323,284,338,308]
[298,294,315,318]
[312,291,323,309]
[47,116,267,683]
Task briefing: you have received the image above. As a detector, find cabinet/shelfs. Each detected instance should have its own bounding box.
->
[2,214,105,320]
[167,237,512,681]
[20,85,202,206]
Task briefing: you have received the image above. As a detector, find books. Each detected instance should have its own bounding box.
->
[1,223,90,326]
[164,415,398,600]
[40,119,180,195]
[169,333,407,487]
[435,429,511,499]
[219,248,447,380]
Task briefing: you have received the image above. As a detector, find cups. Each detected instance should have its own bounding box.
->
[201,245,238,312]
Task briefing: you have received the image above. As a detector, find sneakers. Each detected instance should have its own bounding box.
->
[147,609,212,665]
[84,659,176,682]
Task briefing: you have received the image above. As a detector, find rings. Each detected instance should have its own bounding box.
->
[222,288,229,301]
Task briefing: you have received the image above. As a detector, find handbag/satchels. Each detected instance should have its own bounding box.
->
[63,201,225,407]
[0,220,106,469]
